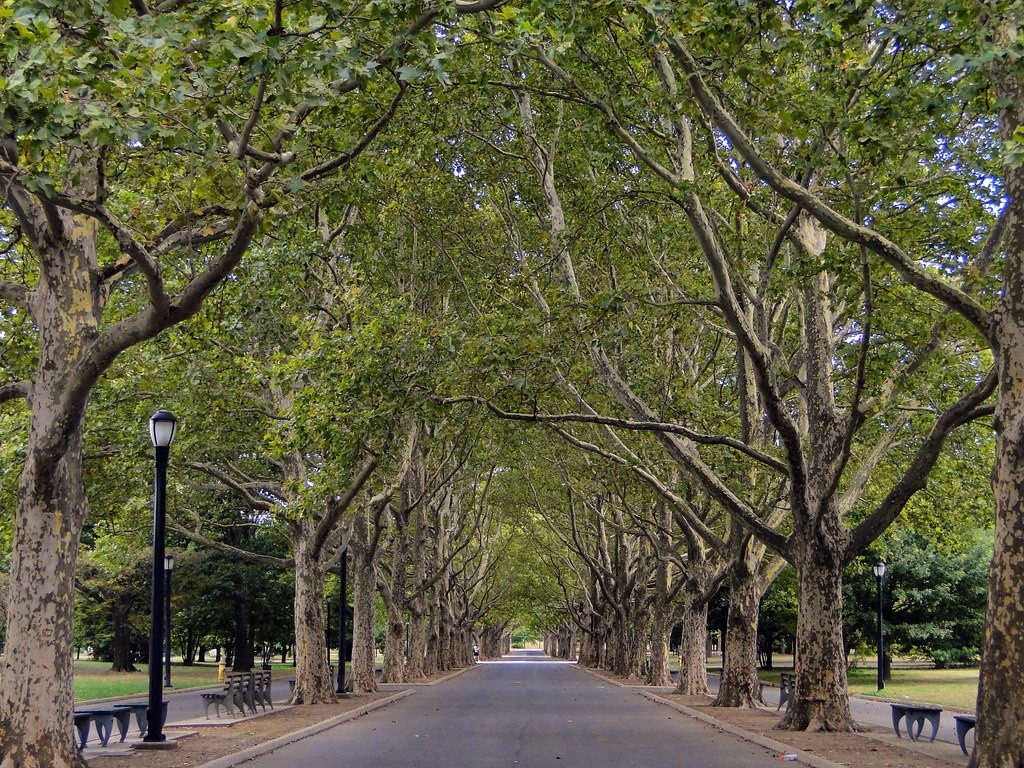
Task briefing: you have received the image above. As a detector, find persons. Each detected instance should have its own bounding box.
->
[473,645,481,662]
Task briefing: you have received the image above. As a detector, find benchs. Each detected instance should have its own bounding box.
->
[889,703,944,743]
[74,706,132,748]
[113,699,170,738]
[777,672,797,711]
[73,713,93,749]
[954,715,975,756]
[201,671,274,719]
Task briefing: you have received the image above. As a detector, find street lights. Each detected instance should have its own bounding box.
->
[130,409,178,752]
[873,561,887,693]
[406,618,409,658]
[335,526,350,699]
[325,595,334,675]
[163,554,174,688]
[719,596,725,670]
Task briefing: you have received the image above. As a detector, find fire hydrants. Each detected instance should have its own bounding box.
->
[216,657,228,682]
